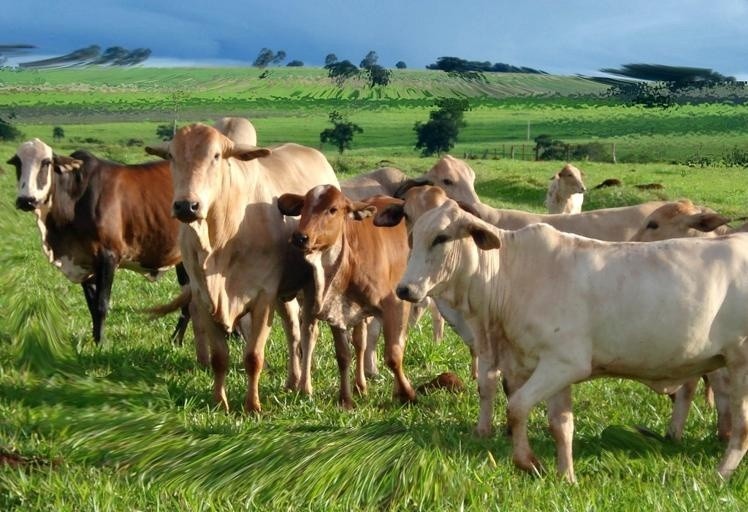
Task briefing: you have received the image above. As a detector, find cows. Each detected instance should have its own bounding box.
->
[372,154,745,486]
[8,116,478,417]
